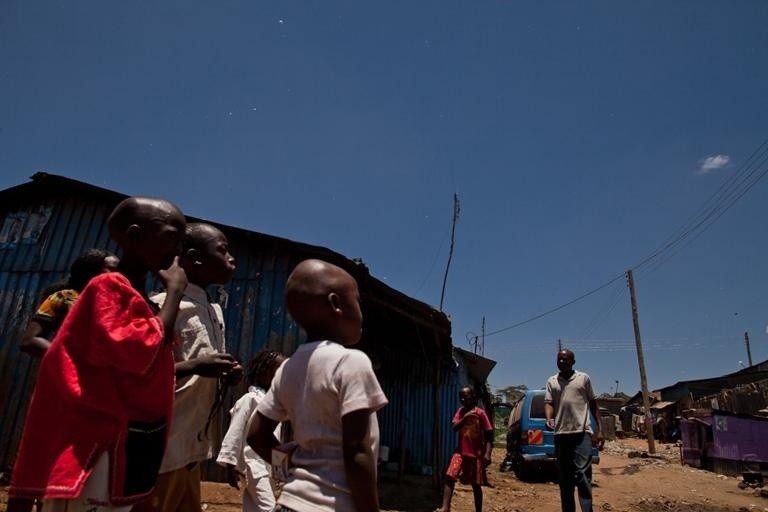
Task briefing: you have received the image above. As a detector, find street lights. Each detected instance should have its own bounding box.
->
[736,360,745,369]
[613,380,619,393]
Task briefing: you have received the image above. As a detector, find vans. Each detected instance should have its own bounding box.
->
[501,387,601,470]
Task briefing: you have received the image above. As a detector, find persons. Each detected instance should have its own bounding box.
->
[247,256,389,512]
[6,249,121,512]
[10,195,188,512]
[544,349,605,512]
[436,385,494,512]
[141,221,242,512]
[656,415,666,444]
[216,349,288,512]
